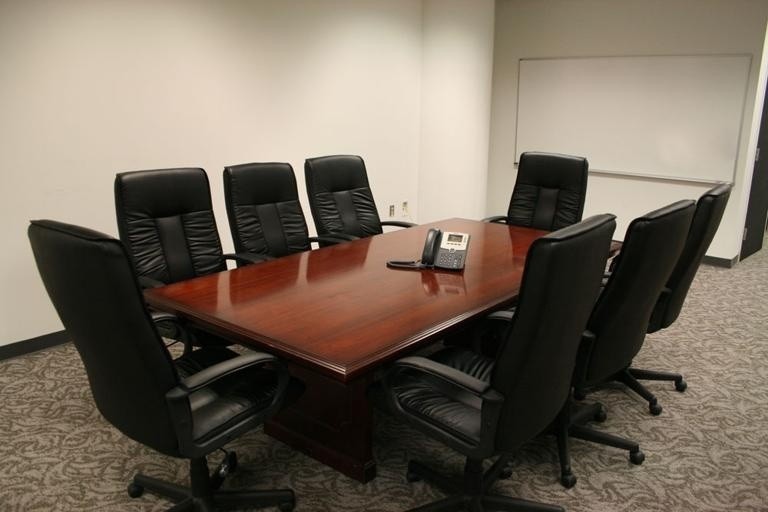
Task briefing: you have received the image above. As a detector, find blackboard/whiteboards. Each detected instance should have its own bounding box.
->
[514,53,753,186]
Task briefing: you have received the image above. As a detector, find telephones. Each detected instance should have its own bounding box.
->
[422,228,471,272]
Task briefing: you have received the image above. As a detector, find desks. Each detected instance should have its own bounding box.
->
[142,218,624,484]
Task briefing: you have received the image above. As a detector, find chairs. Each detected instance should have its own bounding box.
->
[305,153,419,241]
[577,194,706,418]
[223,163,348,262]
[27,220,302,509]
[492,198,700,487]
[116,165,267,351]
[385,215,617,512]
[482,152,589,231]
